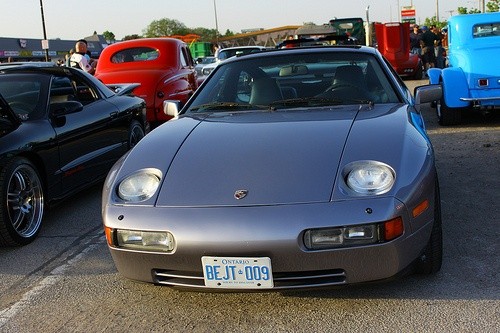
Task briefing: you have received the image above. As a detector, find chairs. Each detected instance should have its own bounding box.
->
[329,65,367,98]
[247,78,284,105]
[35,78,79,103]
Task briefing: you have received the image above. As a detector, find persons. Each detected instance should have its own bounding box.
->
[409,24,448,71]
[56,38,98,74]
[285,33,304,47]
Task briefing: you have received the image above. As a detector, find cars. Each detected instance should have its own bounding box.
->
[0,61,151,248]
[100,46,443,293]
[94,38,199,128]
[427,12,500,125]
